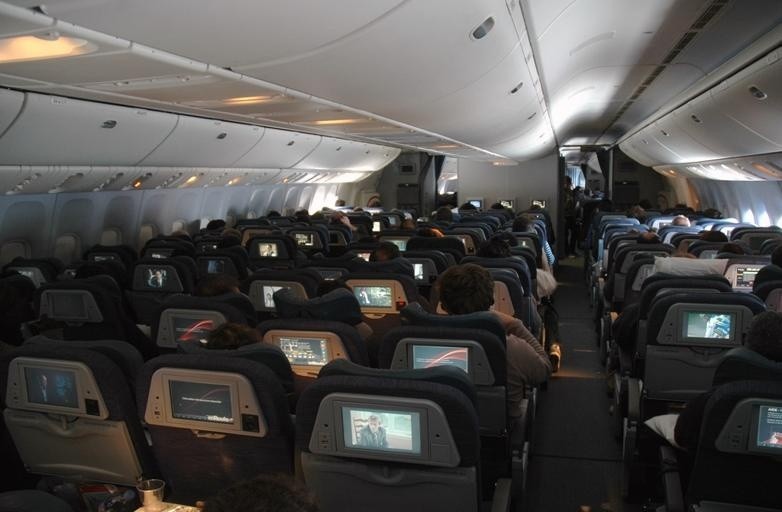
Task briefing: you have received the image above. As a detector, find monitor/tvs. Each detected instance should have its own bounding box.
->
[167,312,218,347]
[202,244,225,275]
[681,308,736,344]
[731,263,765,290]
[0,247,172,416]
[262,284,293,309]
[166,379,233,426]
[351,281,397,311]
[751,402,782,447]
[469,200,482,208]
[257,242,279,258]
[413,263,424,280]
[272,333,330,376]
[336,401,423,455]
[411,342,471,376]
[354,209,438,264]
[532,199,546,209]
[500,200,514,208]
[295,233,314,247]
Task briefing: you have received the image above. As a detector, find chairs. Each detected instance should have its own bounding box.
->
[193,209,546,512]
[1,235,192,511]
[585,208,781,415]
[613,285,781,512]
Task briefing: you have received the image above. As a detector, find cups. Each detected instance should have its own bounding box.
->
[136,477,166,511]
